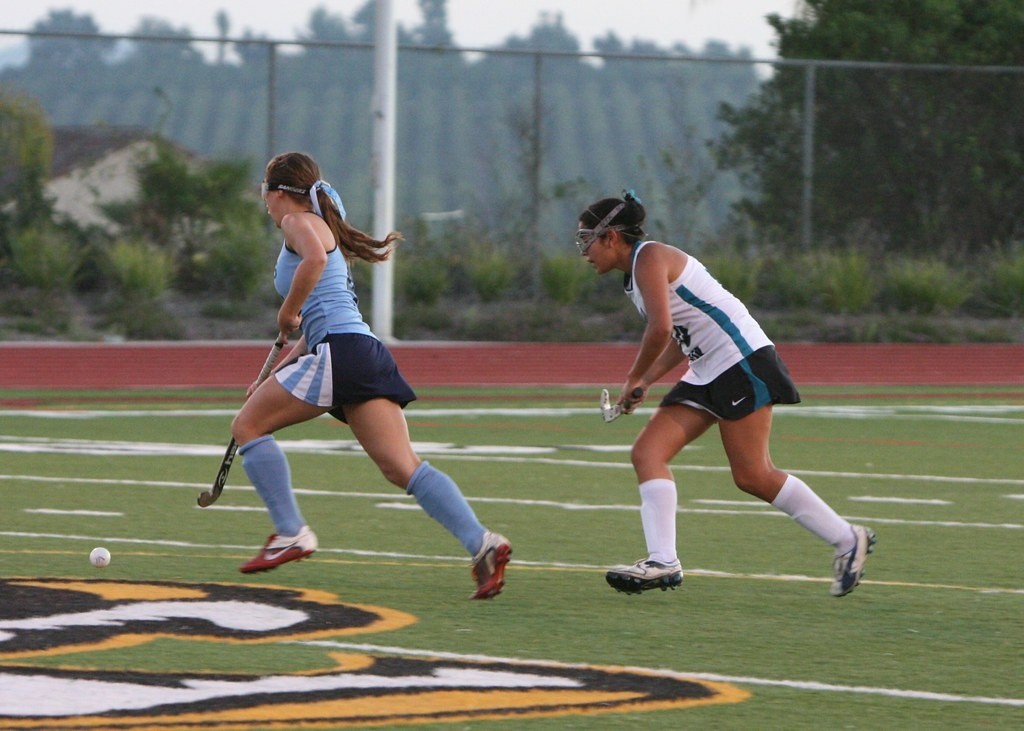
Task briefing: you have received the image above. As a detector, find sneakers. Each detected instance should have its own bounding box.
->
[830,525,878,598]
[604,557,682,595]
[238,524,318,575]
[470,531,511,600]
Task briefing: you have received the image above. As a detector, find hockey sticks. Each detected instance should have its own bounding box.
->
[197,305,301,507]
[600,387,645,422]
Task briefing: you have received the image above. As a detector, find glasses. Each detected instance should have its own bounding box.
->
[574,228,607,255]
[258,182,310,200]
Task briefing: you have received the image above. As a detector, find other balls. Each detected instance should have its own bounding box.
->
[89,546,111,567]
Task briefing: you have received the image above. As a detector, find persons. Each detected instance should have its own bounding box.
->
[577,190,877,597]
[229,149,511,600]
